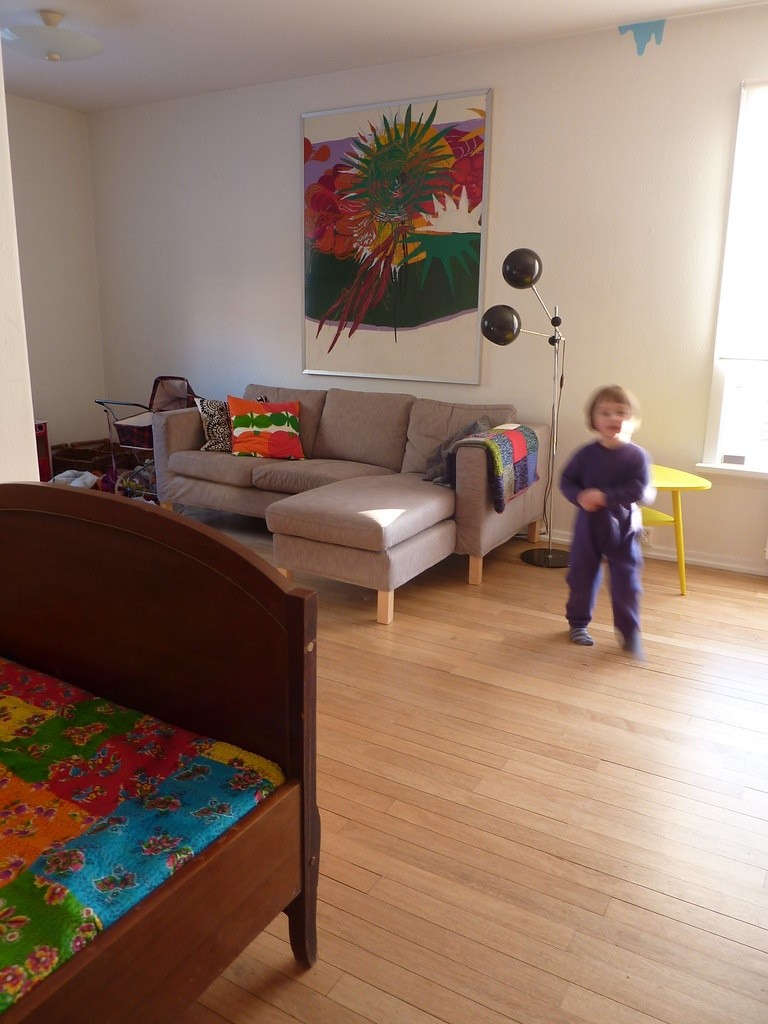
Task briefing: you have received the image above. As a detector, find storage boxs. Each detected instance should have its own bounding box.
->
[50,438,145,476]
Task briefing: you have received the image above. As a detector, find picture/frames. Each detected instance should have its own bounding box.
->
[298,86,495,387]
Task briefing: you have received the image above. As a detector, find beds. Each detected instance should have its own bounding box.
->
[0,481,322,1024]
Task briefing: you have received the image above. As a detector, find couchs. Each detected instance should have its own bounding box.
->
[153,382,549,624]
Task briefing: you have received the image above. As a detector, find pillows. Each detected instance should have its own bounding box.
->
[194,395,267,453]
[227,395,306,460]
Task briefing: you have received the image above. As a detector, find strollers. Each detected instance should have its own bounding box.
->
[95,376,196,516]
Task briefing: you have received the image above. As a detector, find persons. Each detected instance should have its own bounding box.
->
[558,385,649,653]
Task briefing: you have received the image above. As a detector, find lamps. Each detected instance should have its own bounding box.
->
[1,10,103,62]
[480,248,572,568]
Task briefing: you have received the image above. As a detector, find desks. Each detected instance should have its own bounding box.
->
[637,464,714,596]
